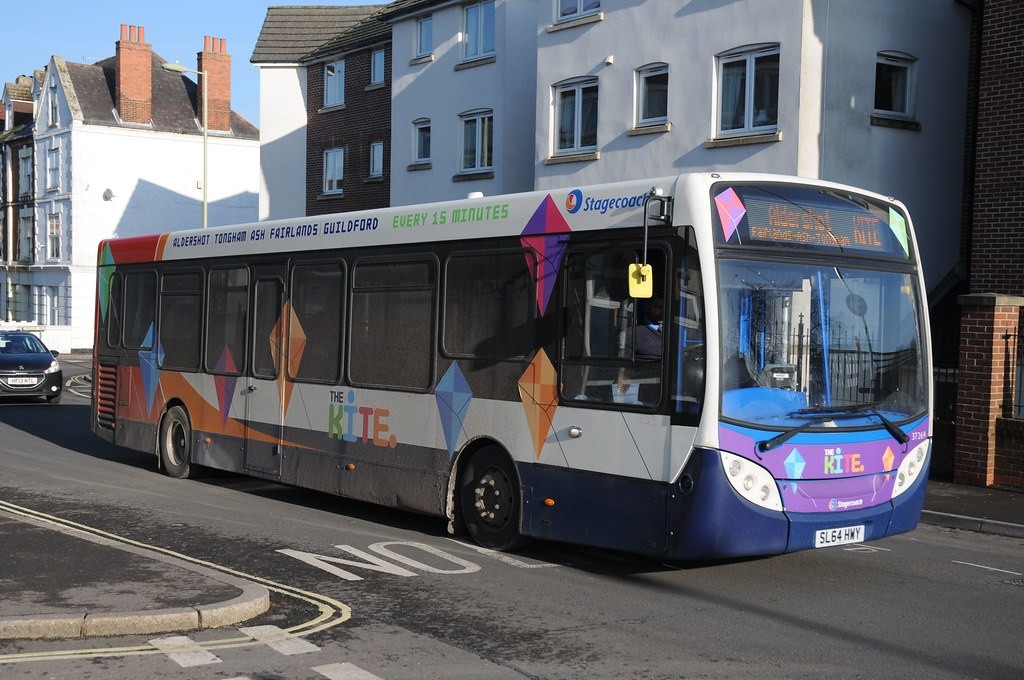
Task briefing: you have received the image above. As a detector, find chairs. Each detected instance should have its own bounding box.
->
[5,339,25,353]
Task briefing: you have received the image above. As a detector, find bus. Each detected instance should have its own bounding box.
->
[90,171,938,574]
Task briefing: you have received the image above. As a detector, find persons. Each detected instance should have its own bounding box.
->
[615,289,664,391]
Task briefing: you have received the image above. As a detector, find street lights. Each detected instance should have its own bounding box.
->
[161,62,208,227]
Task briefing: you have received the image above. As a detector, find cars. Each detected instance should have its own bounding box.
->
[0,332,63,404]
